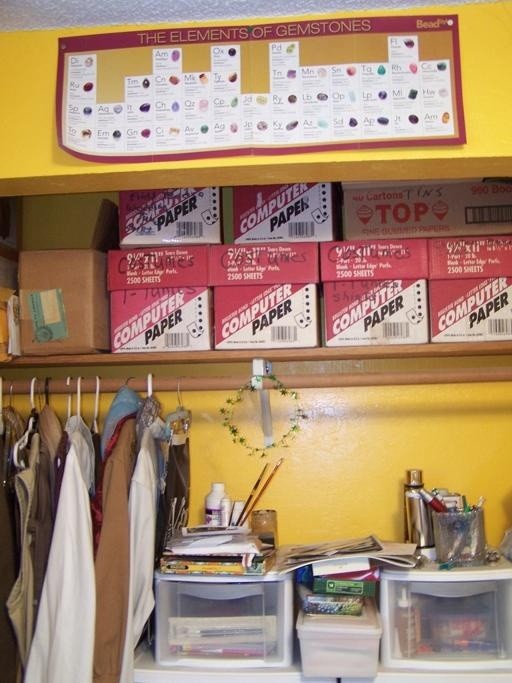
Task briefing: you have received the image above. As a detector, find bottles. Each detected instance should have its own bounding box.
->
[404,469,423,541]
[205,482,231,526]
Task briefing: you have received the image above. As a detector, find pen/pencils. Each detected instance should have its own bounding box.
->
[419,487,486,560]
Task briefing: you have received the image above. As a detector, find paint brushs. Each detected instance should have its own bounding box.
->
[228,458,282,527]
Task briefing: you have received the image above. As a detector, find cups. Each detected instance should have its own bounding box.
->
[251,510,279,550]
[405,493,435,548]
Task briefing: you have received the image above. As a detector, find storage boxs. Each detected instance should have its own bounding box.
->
[337,182,512,242]
[379,552,512,673]
[421,274,507,345]
[154,565,294,668]
[103,234,511,283]
[296,600,383,679]
[315,278,433,348]
[230,183,344,240]
[15,247,109,357]
[114,184,224,246]
[206,283,318,352]
[106,285,214,354]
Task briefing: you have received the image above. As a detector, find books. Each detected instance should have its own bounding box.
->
[266,532,421,575]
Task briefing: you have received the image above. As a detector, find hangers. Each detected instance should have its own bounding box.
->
[0,372,156,475]
